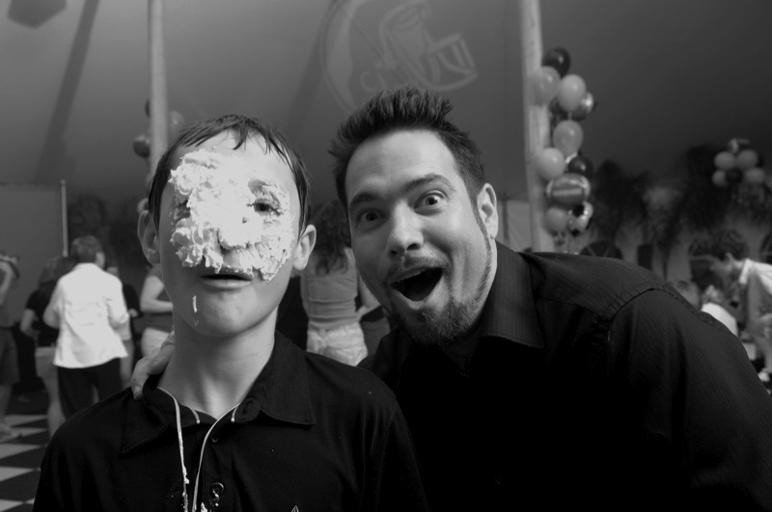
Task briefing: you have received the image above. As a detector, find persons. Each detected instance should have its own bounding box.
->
[3,235,176,439]
[582,223,771,398]
[277,199,390,368]
[32,114,431,511]
[126,84,771,510]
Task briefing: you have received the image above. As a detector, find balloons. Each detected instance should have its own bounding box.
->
[144,99,151,117]
[134,135,150,158]
[530,46,598,243]
[711,138,766,188]
